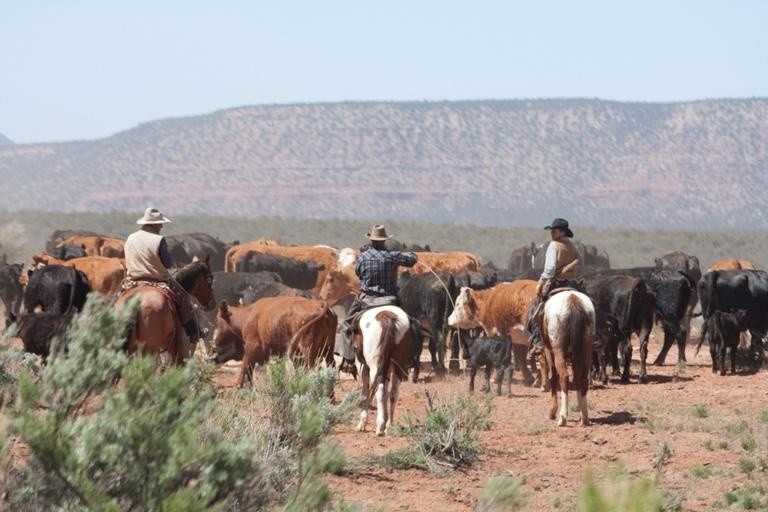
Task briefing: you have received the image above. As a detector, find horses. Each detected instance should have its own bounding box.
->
[111,256,215,369]
[340,303,414,438]
[527,240,596,430]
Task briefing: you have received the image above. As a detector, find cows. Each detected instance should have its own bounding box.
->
[40,256,124,296]
[584,244,597,266]
[596,247,611,270]
[587,273,656,384]
[210,296,346,409]
[459,334,514,397]
[448,279,547,344]
[44,237,88,259]
[44,230,124,258]
[25,264,89,317]
[0,261,25,327]
[710,258,757,352]
[581,266,697,383]
[16,313,69,368]
[163,233,225,270]
[210,270,284,307]
[705,307,751,377]
[319,260,362,309]
[693,270,767,374]
[452,268,498,290]
[572,240,587,268]
[236,249,323,289]
[507,246,531,276]
[223,237,358,298]
[406,254,480,274]
[654,251,703,346]
[401,269,461,384]
[400,242,432,253]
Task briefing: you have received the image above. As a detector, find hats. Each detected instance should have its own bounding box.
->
[544,218,574,238]
[136,207,172,225]
[365,224,393,241]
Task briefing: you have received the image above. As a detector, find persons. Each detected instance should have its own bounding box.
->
[122,207,210,343]
[339,225,418,373]
[525,218,585,392]
